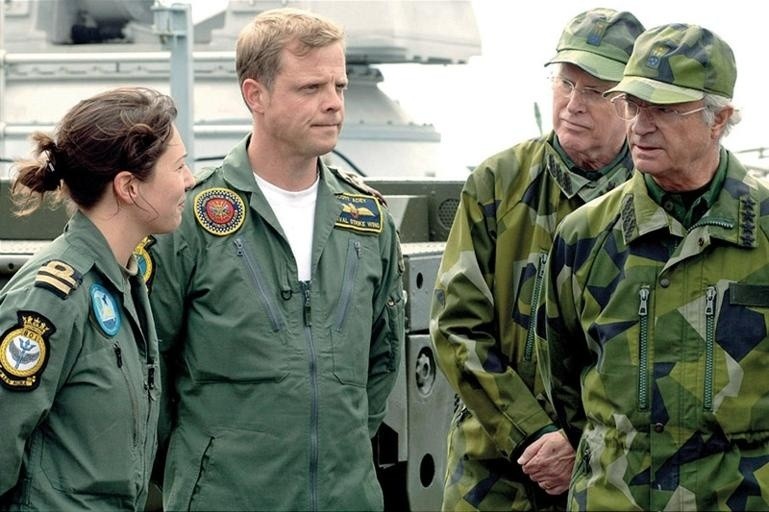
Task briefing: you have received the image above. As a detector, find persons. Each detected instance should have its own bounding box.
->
[149,7,406,512]
[533,25,769,512]
[428,7,646,512]
[0,88,197,512]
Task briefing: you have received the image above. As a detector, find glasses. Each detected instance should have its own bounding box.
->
[612,95,708,121]
[546,75,618,106]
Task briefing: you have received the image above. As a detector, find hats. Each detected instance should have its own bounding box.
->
[545,6,646,85]
[603,23,738,106]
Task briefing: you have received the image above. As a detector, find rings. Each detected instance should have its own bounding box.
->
[544,481,551,491]
